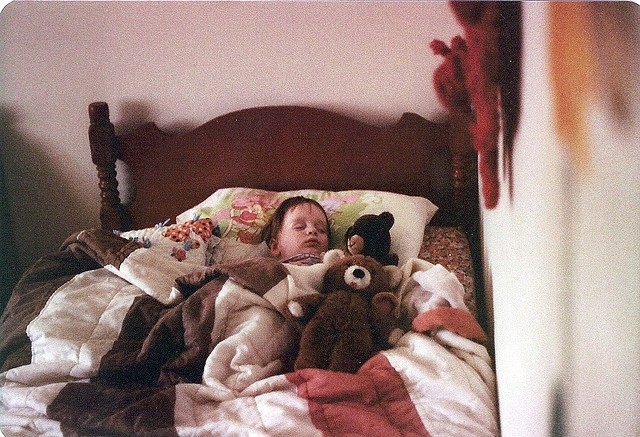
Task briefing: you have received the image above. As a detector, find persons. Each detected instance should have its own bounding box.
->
[262,195,330,265]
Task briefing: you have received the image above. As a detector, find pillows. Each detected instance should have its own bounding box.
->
[197,186,440,269]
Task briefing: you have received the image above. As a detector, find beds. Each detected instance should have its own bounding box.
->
[0,103,494,434]
